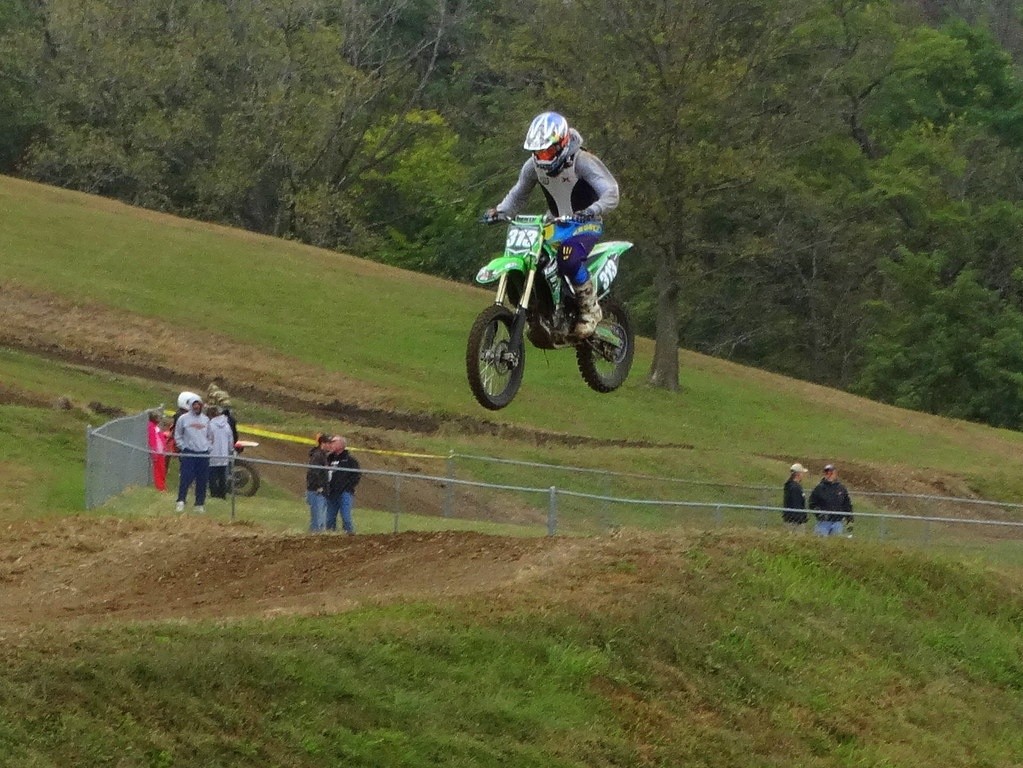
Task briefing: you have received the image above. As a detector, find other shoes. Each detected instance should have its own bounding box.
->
[176,501,185,512]
[192,505,205,513]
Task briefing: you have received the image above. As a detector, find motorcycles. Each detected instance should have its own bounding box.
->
[465,209,635,411]
[228,441,260,496]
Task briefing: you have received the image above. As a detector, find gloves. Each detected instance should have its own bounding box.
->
[482,210,499,226]
[572,208,594,224]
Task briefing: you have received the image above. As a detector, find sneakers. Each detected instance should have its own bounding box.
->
[574,299,603,340]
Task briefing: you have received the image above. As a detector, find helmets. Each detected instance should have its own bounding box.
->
[524,111,570,174]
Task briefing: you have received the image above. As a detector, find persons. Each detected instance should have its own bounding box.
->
[484,112,621,338]
[806,462,855,537]
[146,410,167,490]
[306,434,360,536]
[175,395,215,513]
[165,378,238,499]
[782,464,810,531]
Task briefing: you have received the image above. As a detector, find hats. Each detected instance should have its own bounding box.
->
[823,464,834,472]
[790,463,809,473]
[317,433,335,443]
